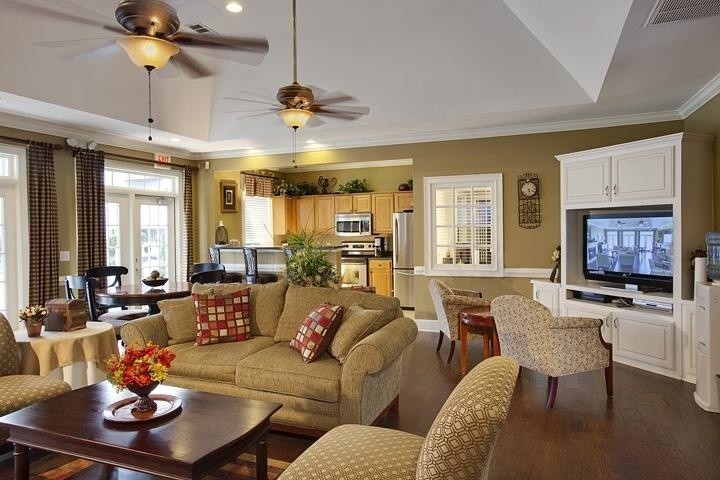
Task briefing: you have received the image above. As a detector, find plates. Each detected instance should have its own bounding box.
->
[103,393,182,426]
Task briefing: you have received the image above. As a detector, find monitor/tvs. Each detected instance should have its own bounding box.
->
[582,212,673,294]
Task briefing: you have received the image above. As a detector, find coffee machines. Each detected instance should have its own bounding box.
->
[374,237,386,257]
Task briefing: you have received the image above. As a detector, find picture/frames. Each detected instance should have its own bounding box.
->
[219,182,239,214]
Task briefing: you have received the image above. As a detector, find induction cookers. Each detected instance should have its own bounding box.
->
[339,251,376,261]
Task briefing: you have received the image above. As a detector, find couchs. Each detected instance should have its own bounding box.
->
[118,279,420,445]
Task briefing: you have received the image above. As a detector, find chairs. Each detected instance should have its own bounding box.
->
[0,311,98,480]
[190,269,227,284]
[428,278,493,365]
[243,246,278,285]
[83,265,130,317]
[271,354,521,480]
[64,274,152,347]
[207,246,243,283]
[489,293,614,411]
[189,262,225,279]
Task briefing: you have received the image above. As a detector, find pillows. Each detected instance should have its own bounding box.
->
[156,280,399,375]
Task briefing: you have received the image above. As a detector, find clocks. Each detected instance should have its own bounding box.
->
[517,171,542,230]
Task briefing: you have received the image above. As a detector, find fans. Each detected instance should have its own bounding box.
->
[0,0,271,83]
[218,1,374,129]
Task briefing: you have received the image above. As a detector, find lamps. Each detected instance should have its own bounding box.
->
[275,108,315,170]
[86,140,98,151]
[114,34,180,144]
[66,137,81,148]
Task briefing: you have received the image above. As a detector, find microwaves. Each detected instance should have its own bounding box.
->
[334,212,372,236]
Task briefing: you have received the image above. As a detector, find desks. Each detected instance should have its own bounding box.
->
[459,305,502,376]
[9,320,122,394]
[210,242,349,289]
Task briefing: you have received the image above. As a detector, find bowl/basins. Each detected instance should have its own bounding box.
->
[142,277,168,287]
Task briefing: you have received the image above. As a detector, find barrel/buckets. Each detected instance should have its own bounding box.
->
[705,231,720,281]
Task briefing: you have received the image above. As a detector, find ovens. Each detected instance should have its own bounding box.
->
[340,259,366,287]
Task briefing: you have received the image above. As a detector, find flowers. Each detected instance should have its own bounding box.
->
[16,303,52,325]
[257,203,345,291]
[94,336,177,394]
[548,238,561,284]
[688,244,709,273]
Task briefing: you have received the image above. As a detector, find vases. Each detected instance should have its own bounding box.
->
[123,376,168,414]
[23,318,43,338]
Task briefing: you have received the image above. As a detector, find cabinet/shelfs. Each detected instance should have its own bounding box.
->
[559,294,681,382]
[553,130,717,210]
[694,280,720,414]
[367,255,395,298]
[272,191,414,237]
[680,297,697,387]
[529,278,561,318]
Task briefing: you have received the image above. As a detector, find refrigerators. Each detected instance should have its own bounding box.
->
[392,211,418,310]
